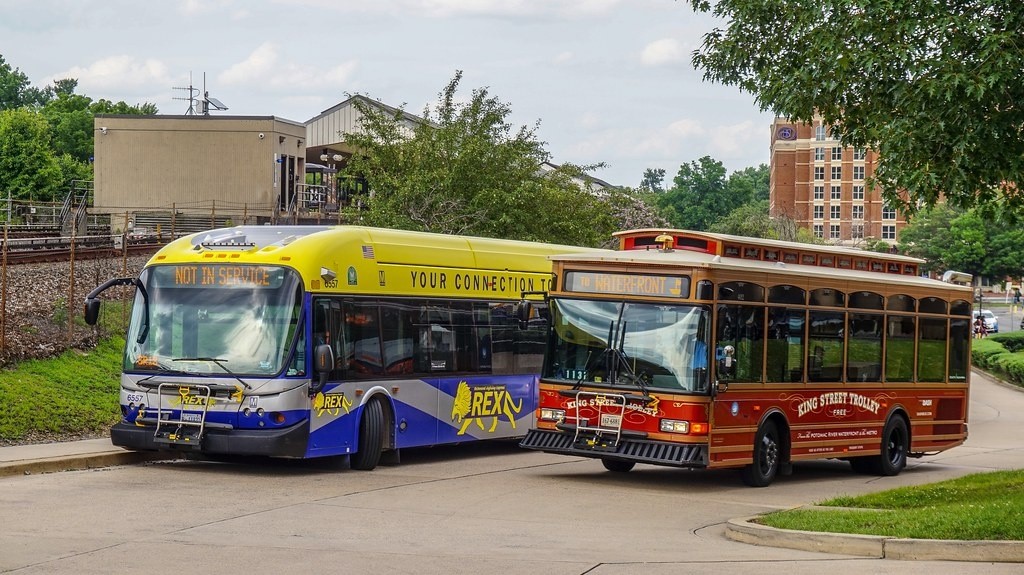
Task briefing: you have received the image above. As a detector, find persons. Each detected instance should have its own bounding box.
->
[648,330,707,371]
[973,316,988,340]
[1014,289,1022,302]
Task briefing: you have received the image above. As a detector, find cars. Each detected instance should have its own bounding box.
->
[972,310,999,334]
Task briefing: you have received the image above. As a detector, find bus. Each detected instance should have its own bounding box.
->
[515,227,974,488]
[83,225,614,472]
